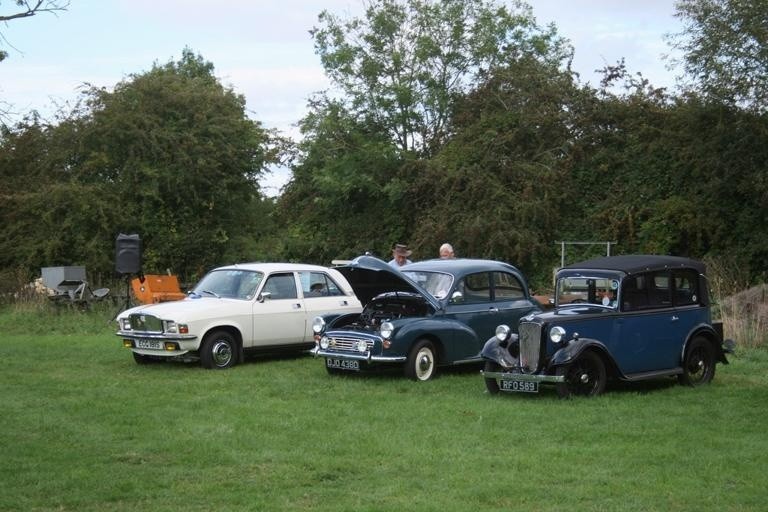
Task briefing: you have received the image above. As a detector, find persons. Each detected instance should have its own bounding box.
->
[387,244,417,284]
[439,243,454,258]
[596,288,615,306]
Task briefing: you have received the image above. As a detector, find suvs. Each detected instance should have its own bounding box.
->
[479,248,736,401]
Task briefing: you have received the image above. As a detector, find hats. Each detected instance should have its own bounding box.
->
[391,243,414,258]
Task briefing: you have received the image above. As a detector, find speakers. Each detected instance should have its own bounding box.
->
[116,238,143,274]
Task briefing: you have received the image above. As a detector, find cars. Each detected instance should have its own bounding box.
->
[111,262,368,375]
[306,247,546,387]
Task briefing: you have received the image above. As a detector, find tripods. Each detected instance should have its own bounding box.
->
[106,274,136,324]
